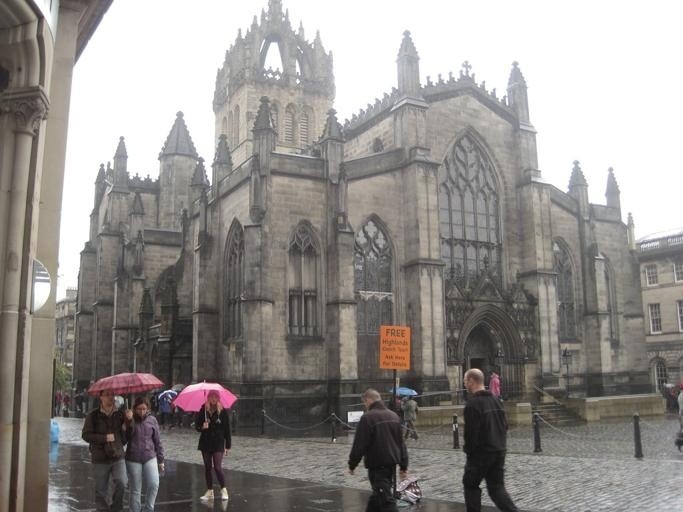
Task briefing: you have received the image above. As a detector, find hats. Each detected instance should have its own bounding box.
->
[207,390,221,399]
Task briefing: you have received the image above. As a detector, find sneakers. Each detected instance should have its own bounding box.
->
[200,489,214,500]
[220,488,229,500]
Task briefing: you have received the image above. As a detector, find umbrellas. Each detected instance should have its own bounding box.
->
[171,384,186,391]
[173,380,238,423]
[88,372,165,409]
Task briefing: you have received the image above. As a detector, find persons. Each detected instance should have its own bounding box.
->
[194,391,232,500]
[122,397,164,512]
[675,384,683,452]
[347,387,418,512]
[55,389,201,429]
[463,368,519,512]
[82,390,133,512]
